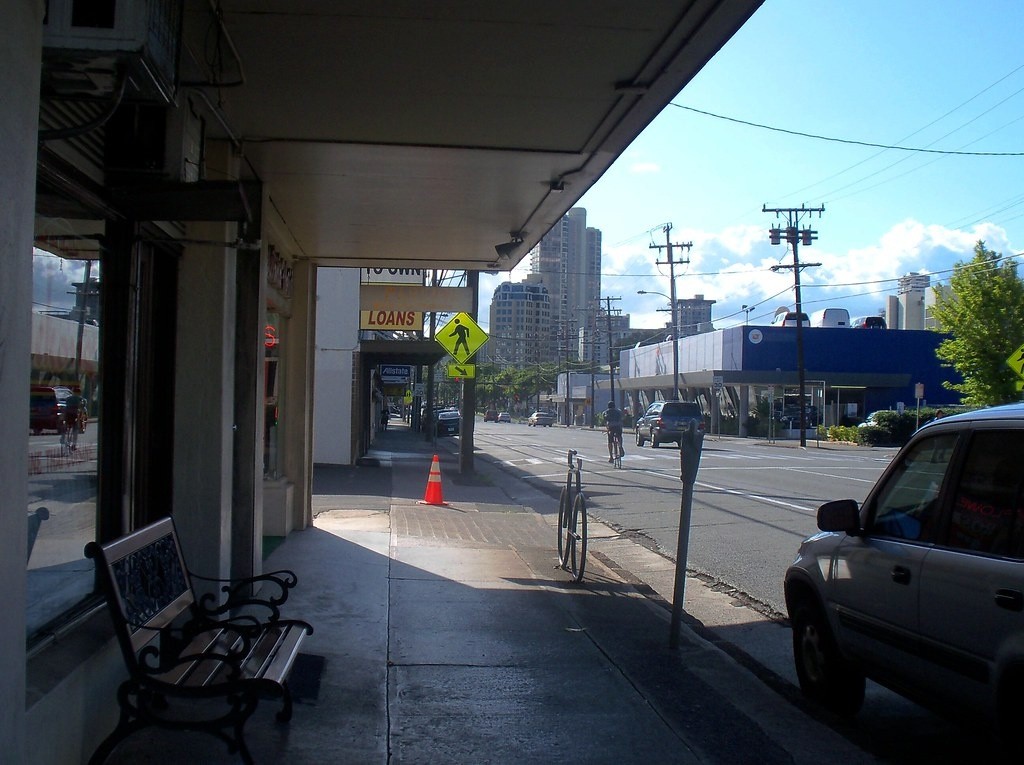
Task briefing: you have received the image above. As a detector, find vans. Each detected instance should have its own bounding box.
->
[770,312,810,327]
[850,316,887,330]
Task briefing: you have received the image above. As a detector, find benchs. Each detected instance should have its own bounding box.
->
[83,510,313,765]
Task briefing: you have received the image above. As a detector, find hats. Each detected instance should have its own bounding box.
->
[607,400,615,407]
[71,385,80,391]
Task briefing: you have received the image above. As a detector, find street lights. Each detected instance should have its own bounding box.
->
[636,289,680,398]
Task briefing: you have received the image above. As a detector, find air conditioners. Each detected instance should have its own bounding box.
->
[42,0,176,109]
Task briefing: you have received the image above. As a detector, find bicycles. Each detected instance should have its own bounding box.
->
[61,419,78,458]
[610,426,622,468]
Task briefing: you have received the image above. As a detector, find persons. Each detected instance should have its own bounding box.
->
[603,401,625,463]
[59,388,88,451]
[380,406,390,431]
[930,410,946,464]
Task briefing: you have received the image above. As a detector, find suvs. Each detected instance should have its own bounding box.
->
[30,386,88,436]
[635,400,705,448]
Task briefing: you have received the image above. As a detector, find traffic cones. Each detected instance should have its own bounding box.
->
[417,455,449,505]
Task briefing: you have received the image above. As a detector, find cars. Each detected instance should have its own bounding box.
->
[784,404,1024,706]
[498,413,511,423]
[419,402,460,435]
[527,410,554,427]
[484,410,498,423]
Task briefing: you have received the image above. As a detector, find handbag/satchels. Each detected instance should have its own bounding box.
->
[387,415,392,420]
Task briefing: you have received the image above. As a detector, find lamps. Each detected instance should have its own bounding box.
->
[495,240,525,260]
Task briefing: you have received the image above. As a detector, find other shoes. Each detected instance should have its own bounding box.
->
[607,459,614,464]
[619,447,624,457]
[71,445,77,451]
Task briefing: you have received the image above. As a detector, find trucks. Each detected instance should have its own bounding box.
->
[810,308,850,328]
